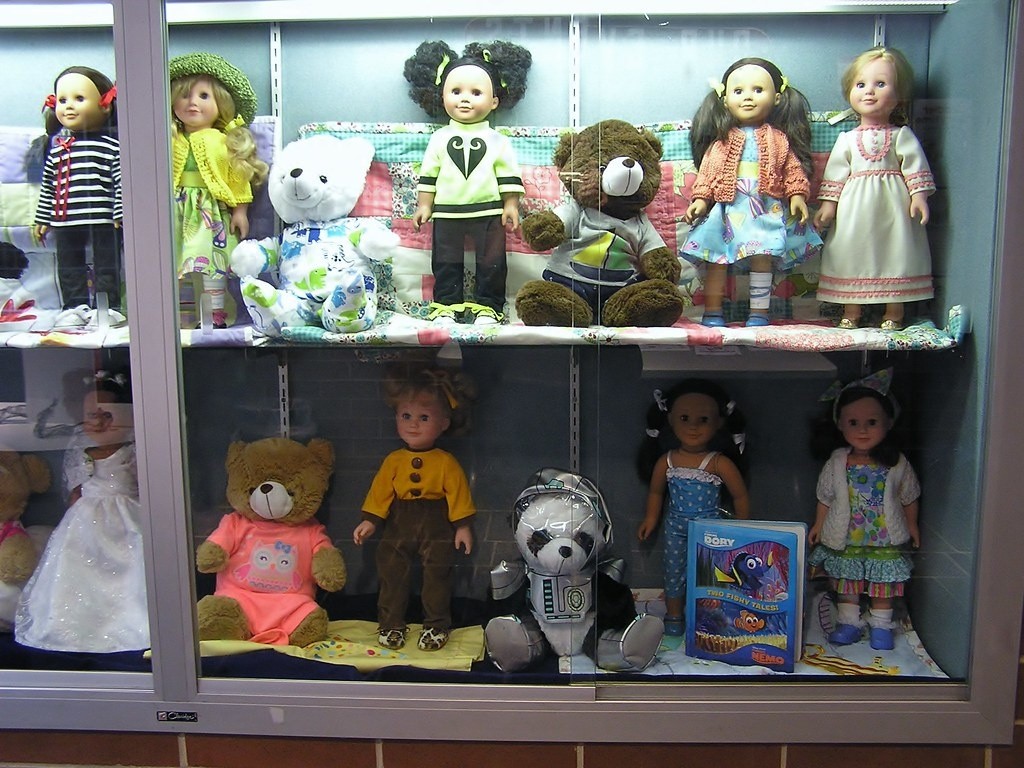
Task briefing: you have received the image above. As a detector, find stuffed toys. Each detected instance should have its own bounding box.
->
[482,468,665,673]
[0,446,51,585]
[514,119,684,328]
[196,438,346,648]
[230,134,400,339]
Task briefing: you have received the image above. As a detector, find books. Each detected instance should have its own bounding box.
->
[685,519,809,673]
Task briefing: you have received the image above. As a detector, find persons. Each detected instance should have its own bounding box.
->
[353,360,477,652]
[34,363,148,653]
[405,38,525,328]
[634,378,751,636]
[806,364,921,650]
[687,56,815,328]
[34,52,268,331]
[811,44,939,331]
